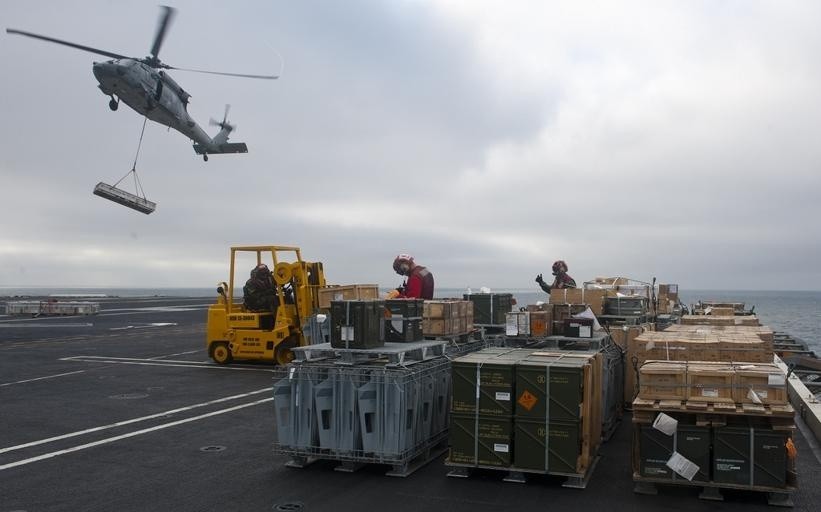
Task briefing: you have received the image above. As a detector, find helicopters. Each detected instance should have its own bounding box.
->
[6,6,281,162]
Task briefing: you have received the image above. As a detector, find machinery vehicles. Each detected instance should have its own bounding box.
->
[206,244,327,369]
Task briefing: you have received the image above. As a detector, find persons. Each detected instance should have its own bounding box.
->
[392,253,435,300]
[241,263,281,331]
[534,259,578,295]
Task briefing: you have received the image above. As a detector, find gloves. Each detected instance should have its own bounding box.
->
[535,274,542,283]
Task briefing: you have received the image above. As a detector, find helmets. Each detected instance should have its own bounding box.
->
[392,254,414,276]
[552,261,568,274]
